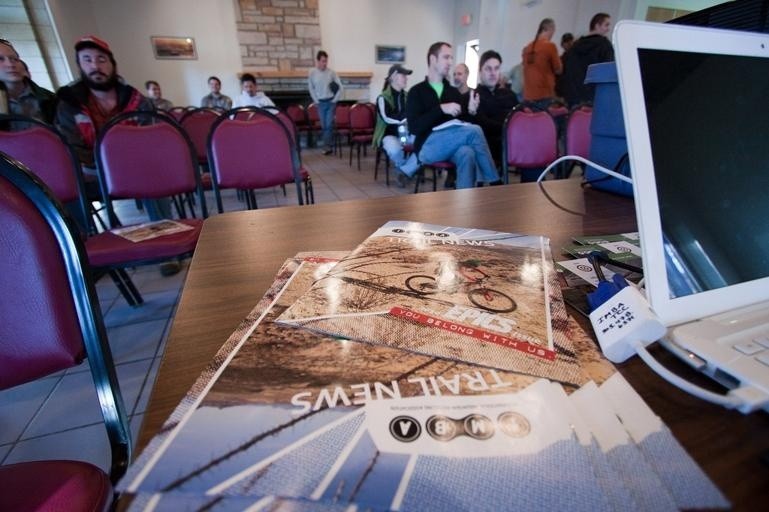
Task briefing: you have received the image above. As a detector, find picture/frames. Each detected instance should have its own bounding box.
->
[375,44,407,65]
[150,36,198,60]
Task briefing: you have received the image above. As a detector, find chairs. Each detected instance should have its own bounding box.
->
[0,150,132,512]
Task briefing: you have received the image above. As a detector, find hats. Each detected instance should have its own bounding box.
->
[389,65,412,76]
[75,35,110,51]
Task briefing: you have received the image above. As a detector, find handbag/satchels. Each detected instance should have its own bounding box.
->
[330,81,340,95]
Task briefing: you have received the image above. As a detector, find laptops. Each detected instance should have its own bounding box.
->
[612,19,769,414]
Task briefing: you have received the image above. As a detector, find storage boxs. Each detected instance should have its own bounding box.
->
[585,60,636,197]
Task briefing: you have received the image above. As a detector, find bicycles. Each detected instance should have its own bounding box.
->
[403,272,517,318]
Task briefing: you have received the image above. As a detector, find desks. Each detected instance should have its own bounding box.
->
[132,175,768,512]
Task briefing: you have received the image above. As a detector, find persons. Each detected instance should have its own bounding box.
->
[371,42,547,190]
[308,50,343,157]
[201,76,232,112]
[0,38,91,234]
[422,259,492,292]
[229,74,279,120]
[144,80,173,113]
[501,12,615,109]
[54,33,183,277]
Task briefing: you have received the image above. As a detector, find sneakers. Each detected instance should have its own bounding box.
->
[160,259,179,276]
[322,147,332,155]
[392,168,406,188]
